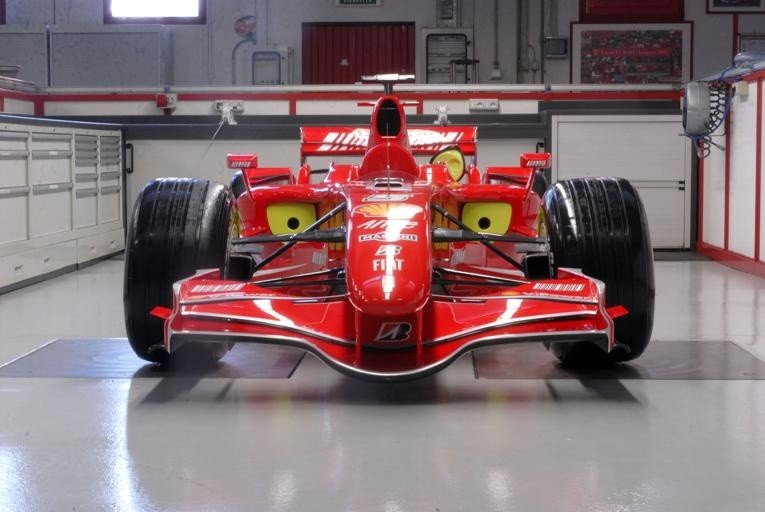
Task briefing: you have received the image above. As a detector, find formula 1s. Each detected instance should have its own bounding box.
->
[123,73,654,384]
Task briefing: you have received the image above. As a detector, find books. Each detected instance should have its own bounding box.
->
[0,66,37,92]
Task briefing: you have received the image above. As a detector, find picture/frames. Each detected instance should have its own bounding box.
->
[569,20,694,86]
[706,0,765,16]
